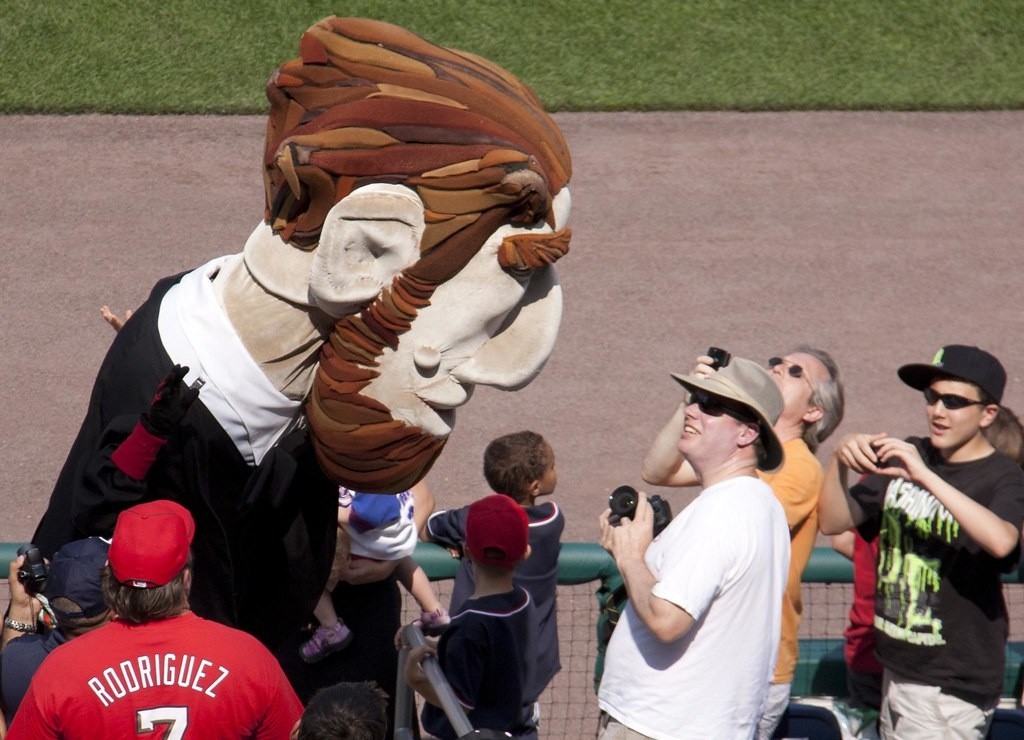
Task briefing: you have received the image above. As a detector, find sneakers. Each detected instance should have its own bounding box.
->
[409,605,451,637]
[300,625,352,664]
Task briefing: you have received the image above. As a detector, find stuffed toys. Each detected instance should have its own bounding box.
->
[1,16,573,688]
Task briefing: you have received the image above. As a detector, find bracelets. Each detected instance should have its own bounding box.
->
[3,618,37,634]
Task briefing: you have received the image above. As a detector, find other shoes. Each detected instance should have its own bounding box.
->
[527,701,542,726]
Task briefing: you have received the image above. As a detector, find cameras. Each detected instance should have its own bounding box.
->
[16,543,50,595]
[607,485,672,536]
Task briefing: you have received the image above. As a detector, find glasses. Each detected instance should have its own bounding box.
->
[924,389,984,411]
[770,356,816,394]
[688,392,747,423]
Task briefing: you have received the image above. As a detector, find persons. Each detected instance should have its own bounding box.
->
[0,306,565,740]
[593,345,1024,740]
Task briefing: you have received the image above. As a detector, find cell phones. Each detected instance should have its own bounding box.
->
[705,346,731,370]
[869,445,888,469]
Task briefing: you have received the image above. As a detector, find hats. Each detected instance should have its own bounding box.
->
[668,358,783,472]
[467,495,529,569]
[44,537,113,617]
[106,500,195,589]
[897,345,1006,403]
